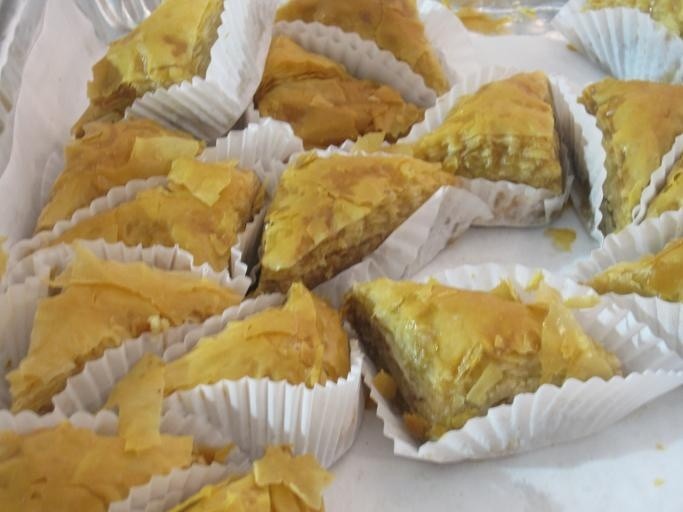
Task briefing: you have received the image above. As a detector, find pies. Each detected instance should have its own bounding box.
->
[0,0,682,512]
